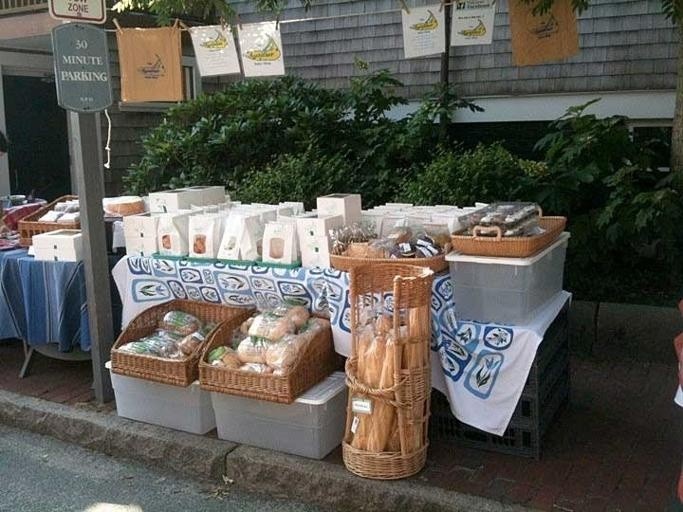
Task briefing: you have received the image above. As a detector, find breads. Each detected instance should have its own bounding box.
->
[115,302,321,376]
[342,316,411,456]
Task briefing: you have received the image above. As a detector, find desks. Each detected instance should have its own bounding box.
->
[0,249,120,378]
[110,254,573,454]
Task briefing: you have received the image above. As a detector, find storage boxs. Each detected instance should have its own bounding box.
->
[210,371,348,459]
[105,361,216,435]
[445,231,571,325]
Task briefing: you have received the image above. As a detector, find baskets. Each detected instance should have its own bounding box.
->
[111,298,339,404]
[341,263,435,480]
[0,195,81,247]
[330,241,453,274]
[449,207,568,257]
